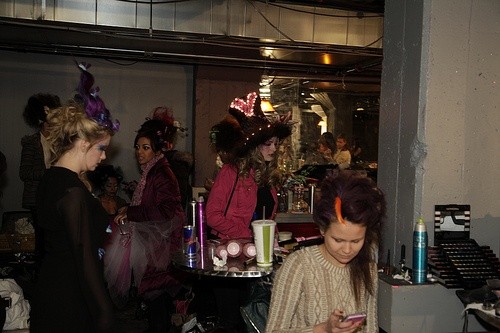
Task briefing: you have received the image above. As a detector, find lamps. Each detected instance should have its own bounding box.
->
[356,100,366,111]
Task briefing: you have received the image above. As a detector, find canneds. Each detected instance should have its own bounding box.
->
[186,258,197,268]
[183,226,196,259]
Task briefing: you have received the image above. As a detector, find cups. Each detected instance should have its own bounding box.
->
[251,219,276,270]
[278,232,292,241]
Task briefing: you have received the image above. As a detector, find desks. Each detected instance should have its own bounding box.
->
[456,290,500,333]
[275,216,319,237]
[171,257,273,278]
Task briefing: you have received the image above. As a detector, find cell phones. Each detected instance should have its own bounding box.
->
[341,314,367,325]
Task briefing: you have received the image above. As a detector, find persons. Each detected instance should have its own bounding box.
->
[86,164,127,252]
[20,95,62,215]
[107,106,185,333]
[311,132,352,172]
[32,90,117,333]
[265,176,386,333]
[201,93,291,333]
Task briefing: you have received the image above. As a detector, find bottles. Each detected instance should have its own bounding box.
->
[243,243,257,259]
[216,244,228,259]
[226,240,243,259]
[190,196,207,252]
[207,240,216,263]
[412,218,427,286]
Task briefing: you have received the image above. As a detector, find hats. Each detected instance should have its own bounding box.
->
[229,90,292,158]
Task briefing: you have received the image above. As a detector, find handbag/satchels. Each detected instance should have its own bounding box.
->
[208,233,221,239]
